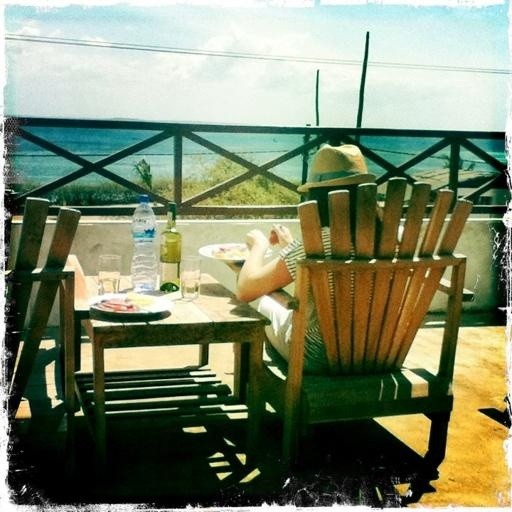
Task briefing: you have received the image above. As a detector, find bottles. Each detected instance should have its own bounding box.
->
[130,194,180,295]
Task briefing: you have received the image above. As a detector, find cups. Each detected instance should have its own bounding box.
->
[97,254,120,294]
[181,256,202,300]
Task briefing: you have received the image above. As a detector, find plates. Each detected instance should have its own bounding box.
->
[199,243,275,261]
[87,293,174,315]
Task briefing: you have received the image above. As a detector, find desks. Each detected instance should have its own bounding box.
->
[74,271,272,458]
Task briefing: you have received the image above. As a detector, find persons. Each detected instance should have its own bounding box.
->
[236,144,405,374]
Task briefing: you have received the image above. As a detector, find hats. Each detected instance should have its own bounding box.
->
[296,142,377,194]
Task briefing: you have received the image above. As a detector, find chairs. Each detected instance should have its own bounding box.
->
[5,191,90,450]
[225,177,475,482]
